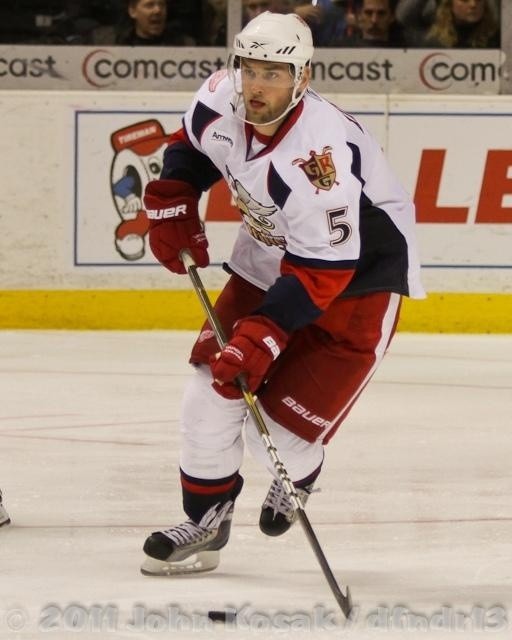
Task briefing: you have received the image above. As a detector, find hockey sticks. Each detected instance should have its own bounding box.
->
[182,252,351,619]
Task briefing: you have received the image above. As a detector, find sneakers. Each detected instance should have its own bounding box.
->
[257,479,315,538]
[115,233,147,256]
[143,502,234,562]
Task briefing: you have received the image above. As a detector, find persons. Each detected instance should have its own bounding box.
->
[0,0,503,50]
[140,10,431,564]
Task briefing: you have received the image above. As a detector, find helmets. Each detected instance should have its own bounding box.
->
[229,10,314,127]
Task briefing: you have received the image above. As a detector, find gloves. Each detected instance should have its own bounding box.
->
[210,314,290,401]
[143,179,211,275]
